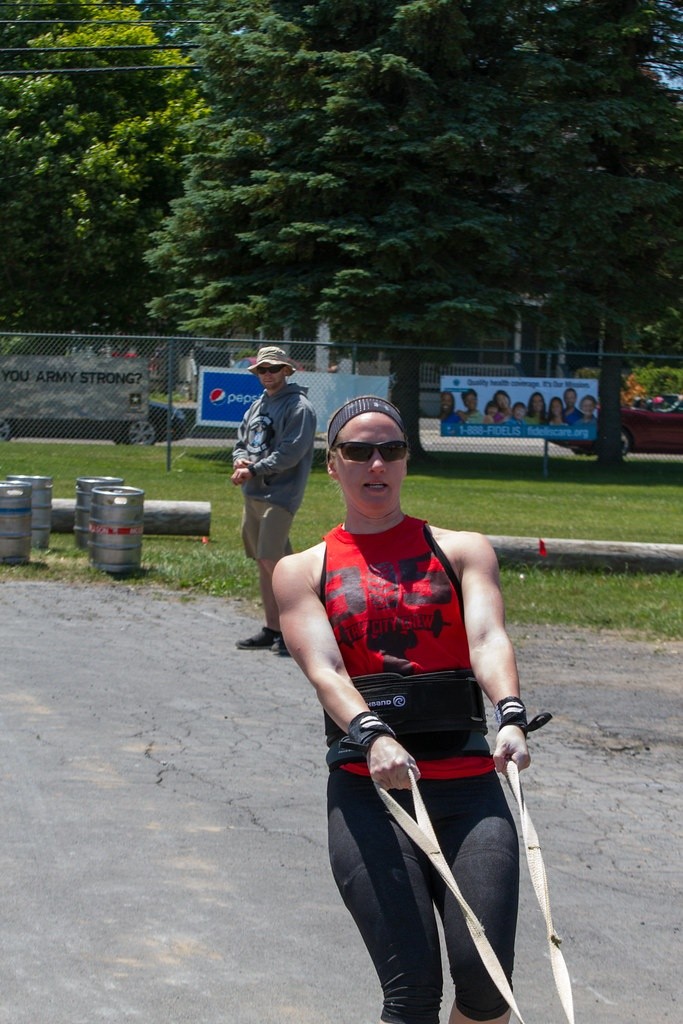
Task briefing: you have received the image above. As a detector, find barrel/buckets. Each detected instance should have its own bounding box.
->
[6,476,54,549]
[0,480,32,566]
[92,485,146,576]
[74,477,124,549]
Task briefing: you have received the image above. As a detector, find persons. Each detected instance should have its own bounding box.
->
[634,397,654,412]
[442,387,596,428]
[651,394,683,414]
[228,346,317,659]
[270,394,534,1022]
[59,342,197,403]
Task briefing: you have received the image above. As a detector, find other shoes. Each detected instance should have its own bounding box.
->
[279,634,290,656]
[235,628,281,648]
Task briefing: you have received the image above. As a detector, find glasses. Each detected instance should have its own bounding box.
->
[334,441,407,462]
[258,364,287,374]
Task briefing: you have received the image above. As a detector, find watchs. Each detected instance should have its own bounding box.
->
[247,464,257,477]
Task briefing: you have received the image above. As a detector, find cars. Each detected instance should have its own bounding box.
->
[546,393,683,455]
[0,402,187,445]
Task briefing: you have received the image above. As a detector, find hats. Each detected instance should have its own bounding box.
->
[247,347,296,376]
